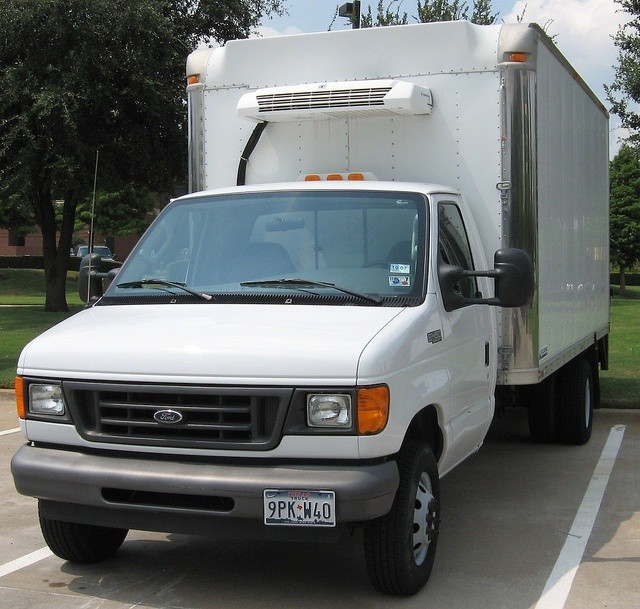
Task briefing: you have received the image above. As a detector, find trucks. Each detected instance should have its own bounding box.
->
[10,20,611,596]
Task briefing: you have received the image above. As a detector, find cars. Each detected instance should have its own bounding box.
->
[70,245,118,262]
